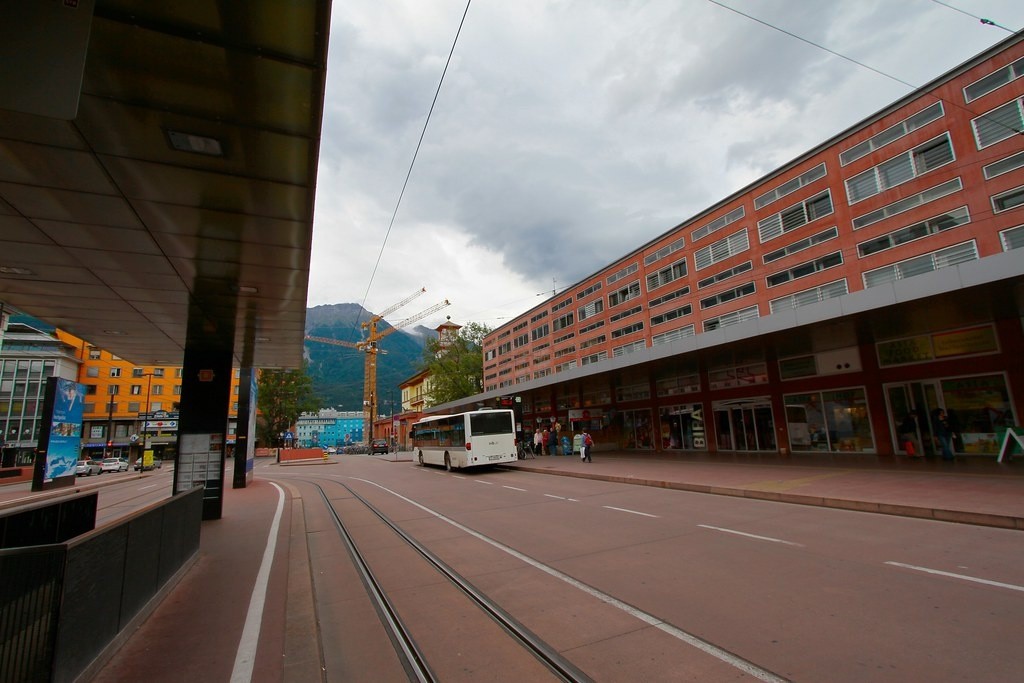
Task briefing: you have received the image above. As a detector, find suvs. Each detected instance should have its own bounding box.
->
[99,457,129,472]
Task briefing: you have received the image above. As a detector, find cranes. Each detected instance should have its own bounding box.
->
[306,286,451,446]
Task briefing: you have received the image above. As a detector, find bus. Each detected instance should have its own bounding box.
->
[409,409,519,472]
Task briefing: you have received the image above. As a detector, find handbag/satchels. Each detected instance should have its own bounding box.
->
[580,447,585,458]
[903,440,916,457]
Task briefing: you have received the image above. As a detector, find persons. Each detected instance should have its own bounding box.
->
[526,433,533,442]
[542,430,551,456]
[533,429,543,456]
[549,428,557,456]
[579,428,594,463]
[902,410,923,460]
[933,408,958,462]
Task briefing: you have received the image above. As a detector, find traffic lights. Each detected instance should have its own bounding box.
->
[107,441,113,452]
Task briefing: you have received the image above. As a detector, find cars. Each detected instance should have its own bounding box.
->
[369,439,390,455]
[133,456,162,472]
[76,460,103,477]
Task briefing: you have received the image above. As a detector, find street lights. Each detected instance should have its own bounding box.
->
[139,373,163,472]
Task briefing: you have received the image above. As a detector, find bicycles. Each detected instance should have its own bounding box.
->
[519,440,535,460]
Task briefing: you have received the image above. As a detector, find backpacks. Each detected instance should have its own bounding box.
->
[583,433,592,446]
